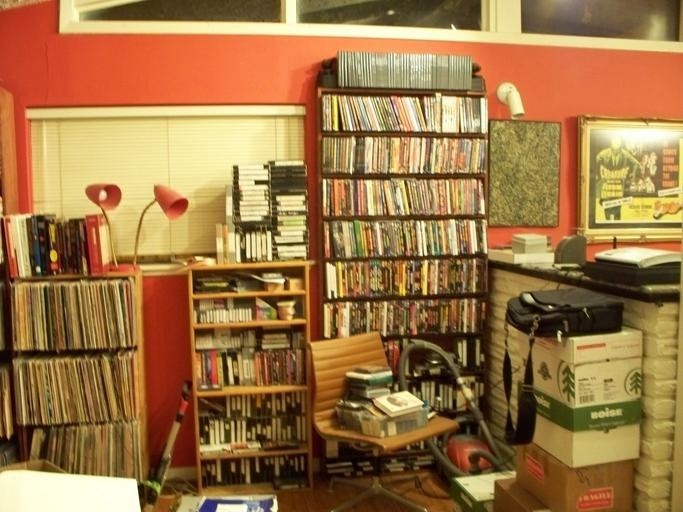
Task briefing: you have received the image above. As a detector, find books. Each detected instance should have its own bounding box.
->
[1,279,143,483]
[332,367,429,437]
[326,428,458,476]
[321,94,484,413]
[3,212,110,279]
[192,270,306,487]
[215,157,308,266]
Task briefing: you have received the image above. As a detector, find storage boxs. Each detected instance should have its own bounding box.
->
[494,325,642,512]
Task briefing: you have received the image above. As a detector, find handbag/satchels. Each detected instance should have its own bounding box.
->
[506,286,624,337]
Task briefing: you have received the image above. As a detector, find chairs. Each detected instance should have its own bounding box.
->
[307,331,458,512]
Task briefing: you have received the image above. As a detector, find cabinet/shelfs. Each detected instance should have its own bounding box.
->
[5,270,149,484]
[188,261,315,498]
[306,88,491,492]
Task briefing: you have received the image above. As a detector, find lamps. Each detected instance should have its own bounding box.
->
[86,184,129,272]
[128,184,189,272]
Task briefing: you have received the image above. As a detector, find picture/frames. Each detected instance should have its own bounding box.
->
[576,114,683,245]
[487,117,562,228]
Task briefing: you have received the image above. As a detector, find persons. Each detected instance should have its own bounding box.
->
[597,134,658,221]
